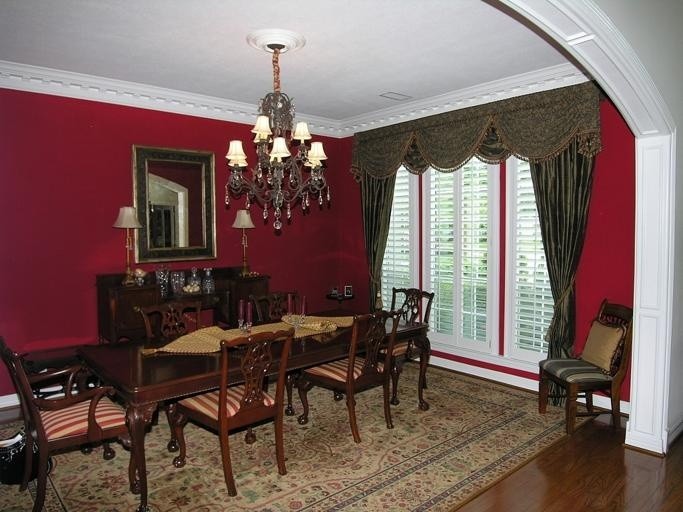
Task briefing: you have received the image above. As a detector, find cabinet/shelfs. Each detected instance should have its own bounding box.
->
[93,266,271,343]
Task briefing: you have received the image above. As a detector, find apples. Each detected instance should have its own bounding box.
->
[135,268,145,276]
[182,284,200,293]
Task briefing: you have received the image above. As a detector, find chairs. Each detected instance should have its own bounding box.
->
[247,291,308,417]
[171,327,295,498]
[537,298,632,436]
[132,300,202,453]
[296,309,403,443]
[0,335,143,511]
[374,287,435,412]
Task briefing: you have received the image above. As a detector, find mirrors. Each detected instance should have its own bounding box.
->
[130,143,217,265]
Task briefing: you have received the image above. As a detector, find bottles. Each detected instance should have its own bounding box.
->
[201,267,215,295]
[187,265,201,285]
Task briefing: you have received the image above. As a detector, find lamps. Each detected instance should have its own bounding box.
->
[230,209,256,276]
[224,43,330,231]
[110,206,143,285]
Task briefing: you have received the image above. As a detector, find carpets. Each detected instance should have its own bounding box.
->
[0,355,601,512]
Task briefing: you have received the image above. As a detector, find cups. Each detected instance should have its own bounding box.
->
[169,271,184,294]
[153,263,171,295]
